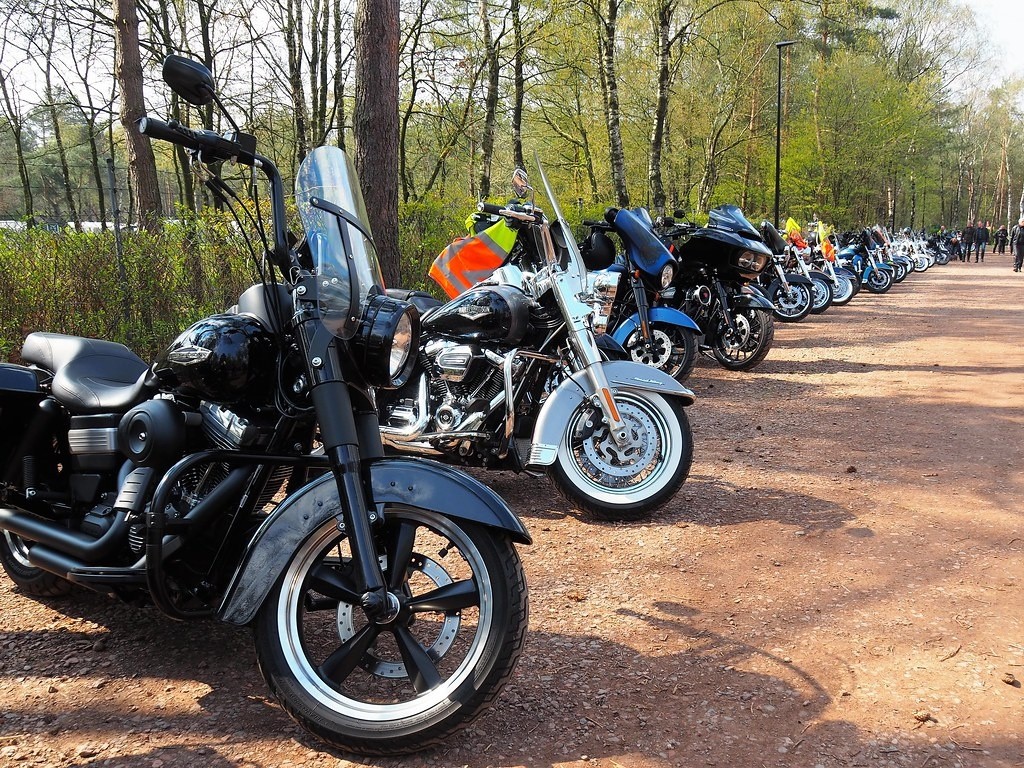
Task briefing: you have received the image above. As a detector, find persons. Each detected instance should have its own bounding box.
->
[939,225,1008,260]
[974,219,989,263]
[961,220,976,262]
[1010,218,1024,272]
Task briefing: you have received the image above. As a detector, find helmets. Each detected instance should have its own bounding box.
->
[904,227,911,235]
[579,232,616,271]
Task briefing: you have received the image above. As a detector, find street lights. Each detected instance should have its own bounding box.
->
[773,39,797,229]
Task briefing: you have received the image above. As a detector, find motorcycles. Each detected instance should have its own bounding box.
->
[332,156,981,524]
[0,53,534,758]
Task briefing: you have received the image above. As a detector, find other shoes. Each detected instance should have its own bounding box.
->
[1014,268,1018,272]
[981,259,983,262]
[1019,269,1021,272]
[962,259,965,262]
[967,259,970,262]
[975,260,978,263]
